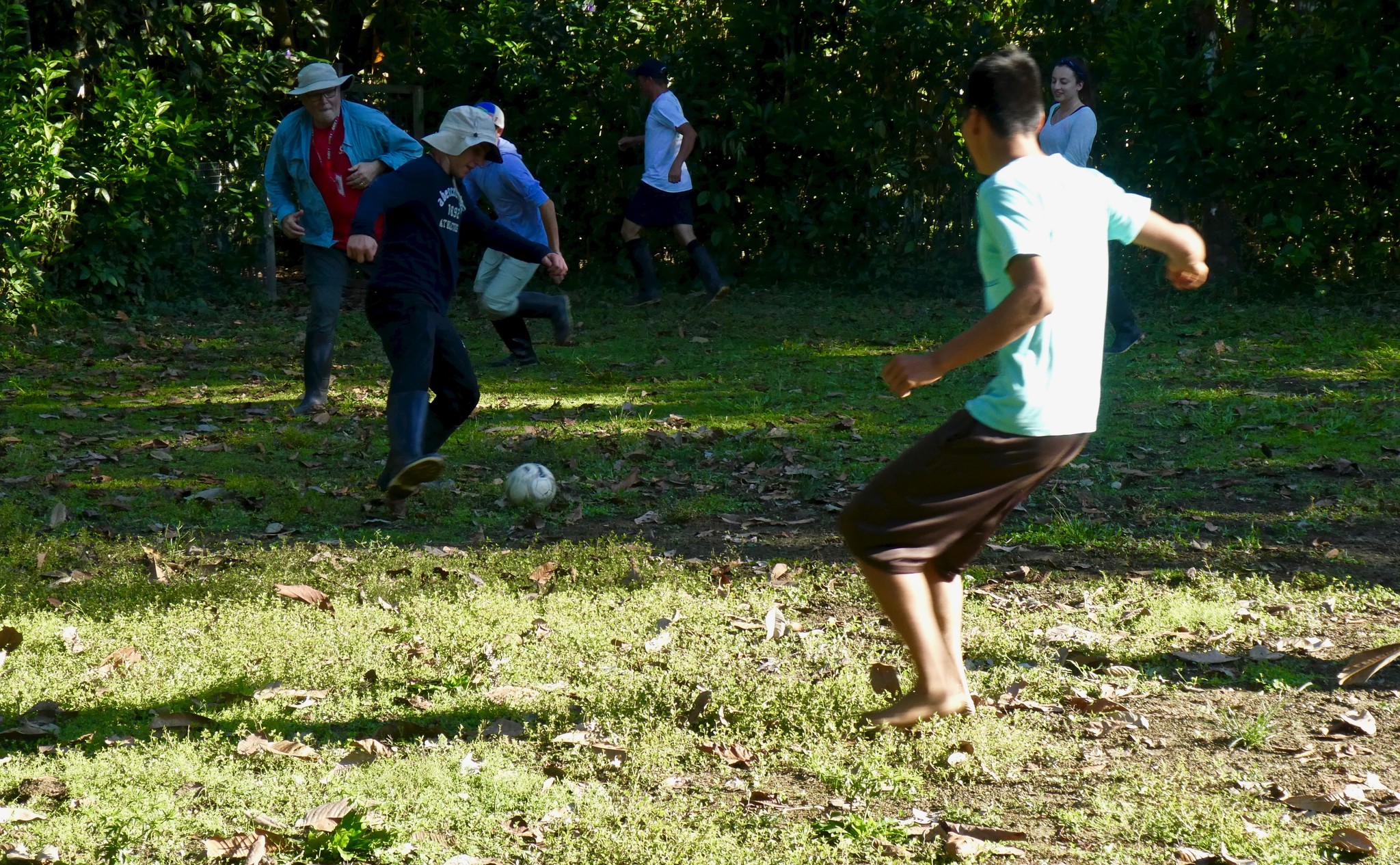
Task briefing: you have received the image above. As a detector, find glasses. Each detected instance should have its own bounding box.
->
[304,87,337,102]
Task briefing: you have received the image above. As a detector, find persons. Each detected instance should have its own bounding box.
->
[839,51,1208,727]
[263,63,424,415]
[461,102,574,366]
[1039,58,1144,353]
[345,105,568,501]
[618,58,729,307]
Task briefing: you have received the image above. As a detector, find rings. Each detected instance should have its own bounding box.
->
[361,182,363,186]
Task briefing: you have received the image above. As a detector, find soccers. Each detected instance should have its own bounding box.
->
[507,463,557,510]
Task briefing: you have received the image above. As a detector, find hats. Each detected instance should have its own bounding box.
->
[421,103,503,163]
[628,58,668,79]
[285,63,355,95]
[474,101,505,129]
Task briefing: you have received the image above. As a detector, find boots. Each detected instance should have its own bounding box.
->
[620,248,660,308]
[386,391,444,501]
[687,244,730,314]
[1103,284,1145,354]
[376,402,454,514]
[487,311,539,368]
[517,291,573,345]
[285,332,334,417]
